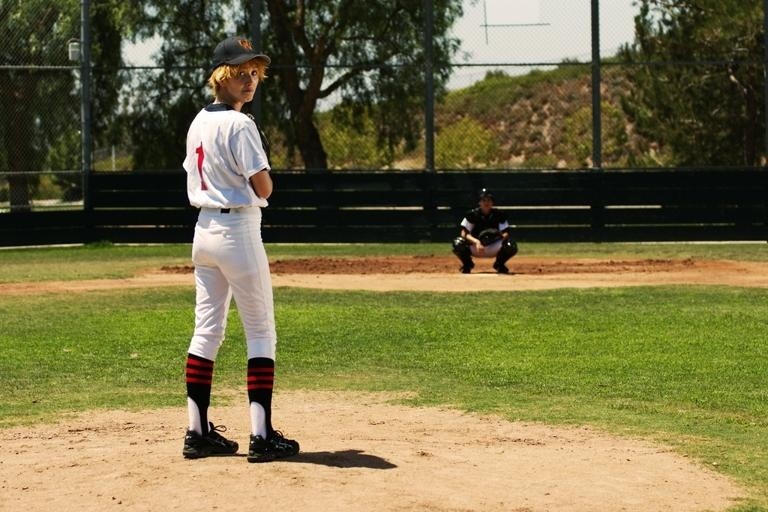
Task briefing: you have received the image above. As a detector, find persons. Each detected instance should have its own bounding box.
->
[179,37,300,463]
[453,187,518,274]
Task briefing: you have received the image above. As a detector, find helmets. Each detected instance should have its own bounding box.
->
[478,188,495,200]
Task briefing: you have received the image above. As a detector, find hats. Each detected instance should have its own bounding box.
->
[213,36,271,69]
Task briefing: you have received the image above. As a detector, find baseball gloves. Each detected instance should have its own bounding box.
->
[480,229,503,246]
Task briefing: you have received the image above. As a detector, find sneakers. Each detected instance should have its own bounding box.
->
[493,262,508,272]
[247,429,299,463]
[183,422,239,459]
[460,261,474,273]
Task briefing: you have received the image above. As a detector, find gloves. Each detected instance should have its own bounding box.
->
[246,112,271,167]
